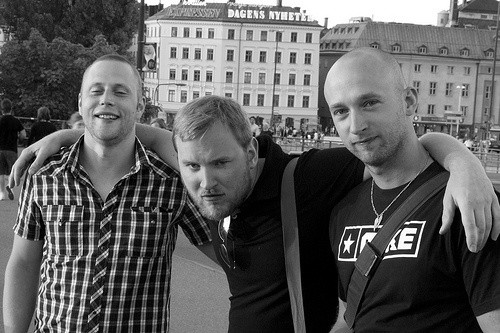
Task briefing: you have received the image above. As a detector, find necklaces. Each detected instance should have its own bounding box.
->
[371,150,430,231]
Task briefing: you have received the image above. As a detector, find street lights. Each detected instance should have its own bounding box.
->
[470,58,481,140]
[154,83,187,104]
[456,85,466,139]
[271,29,285,125]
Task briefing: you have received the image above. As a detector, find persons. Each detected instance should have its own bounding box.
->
[256,116,337,149]
[0,98,26,201]
[456,134,500,155]
[9,95,500,333]
[2,54,220,333]
[27,106,57,148]
[323,47,500,333]
[249,116,260,137]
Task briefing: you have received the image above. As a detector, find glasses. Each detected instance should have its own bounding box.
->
[218,215,236,271]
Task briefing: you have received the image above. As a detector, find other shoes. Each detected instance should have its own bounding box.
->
[0,192,3,200]
[5,184,15,200]
